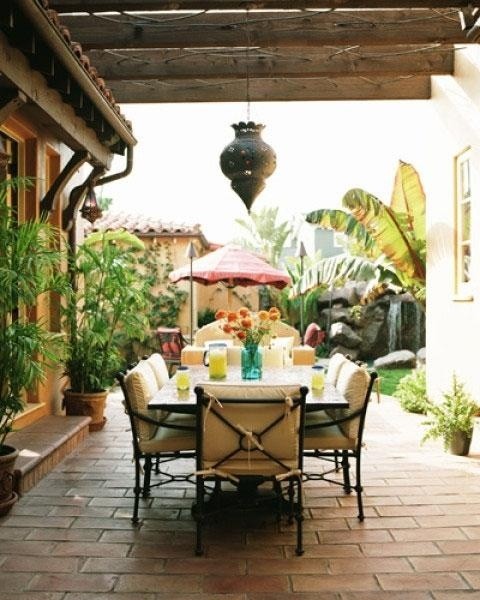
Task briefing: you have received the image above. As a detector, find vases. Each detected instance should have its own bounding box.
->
[241,341,261,380]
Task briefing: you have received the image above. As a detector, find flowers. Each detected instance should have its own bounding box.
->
[214,305,281,361]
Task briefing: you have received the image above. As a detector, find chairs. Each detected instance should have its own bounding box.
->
[262,348,284,368]
[227,346,243,368]
[271,335,293,351]
[301,322,324,349]
[194,385,305,556]
[303,362,377,522]
[118,361,196,522]
[292,347,315,366]
[147,353,198,421]
[158,327,189,379]
[305,353,349,418]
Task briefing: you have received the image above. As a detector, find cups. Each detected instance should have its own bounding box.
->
[312,366,324,390]
[204,343,227,380]
[176,366,189,390]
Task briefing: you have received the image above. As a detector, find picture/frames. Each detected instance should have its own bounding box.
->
[454,144,473,301]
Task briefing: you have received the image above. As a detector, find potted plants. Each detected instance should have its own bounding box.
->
[421,371,480,456]
[61,227,153,431]
[0,158,74,519]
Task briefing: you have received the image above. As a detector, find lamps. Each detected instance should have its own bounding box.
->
[219,0,277,217]
[78,182,102,227]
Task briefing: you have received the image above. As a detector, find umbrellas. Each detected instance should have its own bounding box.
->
[168,244,292,313]
